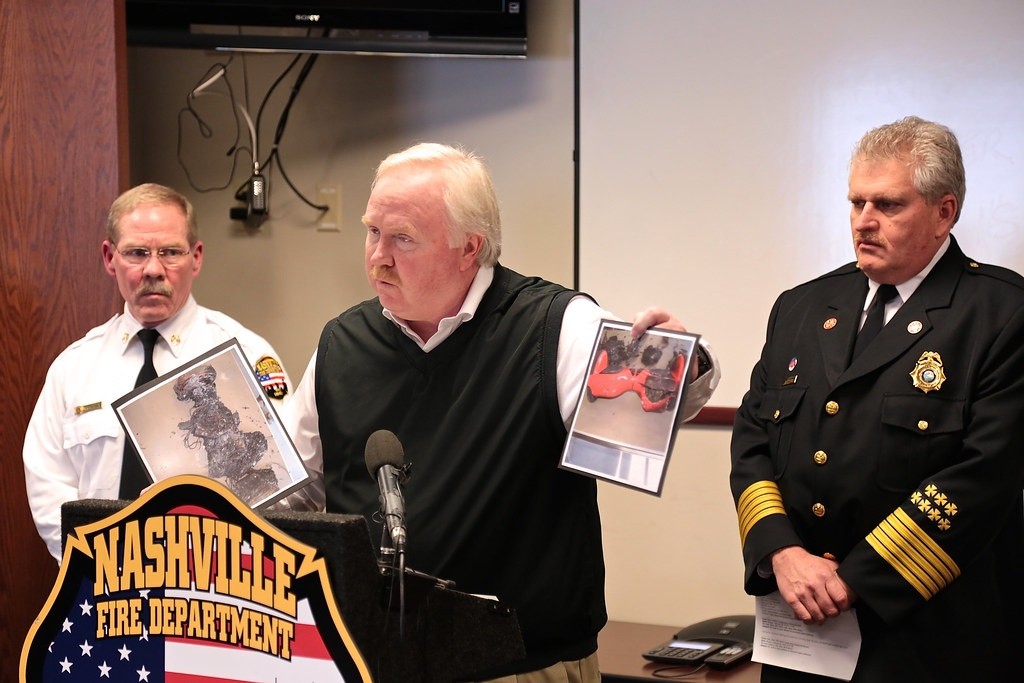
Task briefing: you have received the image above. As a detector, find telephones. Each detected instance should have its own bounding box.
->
[641,636,753,670]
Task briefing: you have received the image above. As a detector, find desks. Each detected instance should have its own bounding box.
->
[596,620,762,683]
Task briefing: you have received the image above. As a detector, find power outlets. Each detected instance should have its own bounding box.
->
[315,181,342,231]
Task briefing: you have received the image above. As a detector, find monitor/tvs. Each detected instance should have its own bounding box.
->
[123,0,529,59]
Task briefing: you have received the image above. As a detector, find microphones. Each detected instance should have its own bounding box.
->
[365,430,408,555]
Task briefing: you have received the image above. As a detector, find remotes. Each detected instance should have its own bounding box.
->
[705,643,753,669]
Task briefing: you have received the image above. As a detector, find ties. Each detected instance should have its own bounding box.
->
[119,329,160,500]
[850,284,899,365]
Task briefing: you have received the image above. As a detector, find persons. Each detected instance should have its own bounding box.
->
[729,117,1024,683]
[261,139,722,682]
[20,181,296,569]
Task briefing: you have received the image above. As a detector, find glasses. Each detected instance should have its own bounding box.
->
[109,236,194,264]
